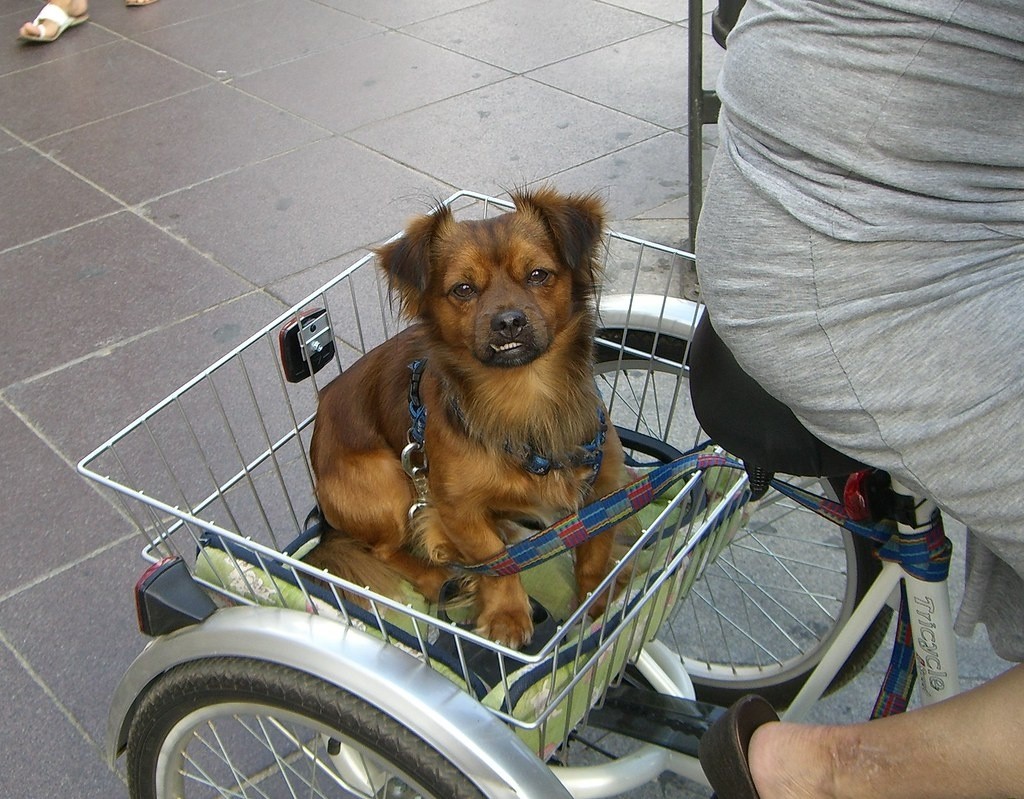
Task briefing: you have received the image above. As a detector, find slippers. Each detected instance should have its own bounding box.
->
[697,694,781,799]
[16,2,90,42]
[124,0,158,6]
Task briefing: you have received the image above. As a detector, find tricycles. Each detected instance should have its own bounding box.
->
[77,188,958,799]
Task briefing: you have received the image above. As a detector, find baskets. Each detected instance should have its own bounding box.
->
[77,187,774,766]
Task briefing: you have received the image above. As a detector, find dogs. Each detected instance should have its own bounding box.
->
[294,175,633,652]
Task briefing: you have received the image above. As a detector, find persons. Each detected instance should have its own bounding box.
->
[694,0,1024,799]
[19,0,157,42]
